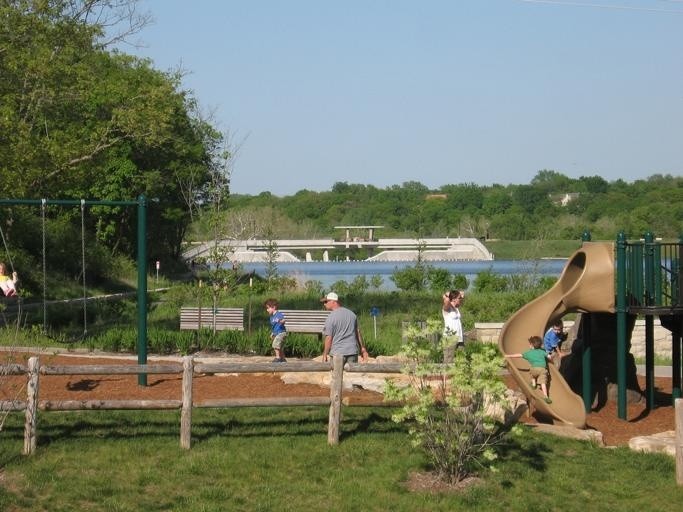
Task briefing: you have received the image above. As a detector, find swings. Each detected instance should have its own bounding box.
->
[0,227,19,305]
[42,199,87,344]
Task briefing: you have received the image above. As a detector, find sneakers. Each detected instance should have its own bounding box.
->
[269,357,286,363]
[530,382,535,390]
[543,397,552,403]
[4,289,16,296]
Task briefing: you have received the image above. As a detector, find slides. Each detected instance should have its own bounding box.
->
[497,241,616,429]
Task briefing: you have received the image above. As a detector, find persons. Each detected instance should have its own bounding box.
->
[504,336,555,404]
[318,292,368,363]
[0,261,19,298]
[440,289,464,364]
[543,319,567,371]
[264,298,287,363]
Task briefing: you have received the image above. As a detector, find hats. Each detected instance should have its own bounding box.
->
[320,291,339,303]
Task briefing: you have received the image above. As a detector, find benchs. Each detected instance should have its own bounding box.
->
[278,309,332,340]
[180,307,245,333]
